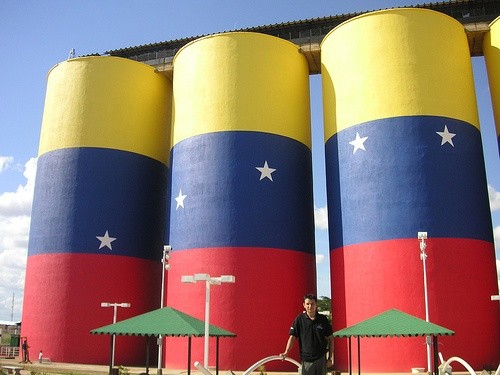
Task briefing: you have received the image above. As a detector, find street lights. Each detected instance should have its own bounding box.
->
[418,231,433,375]
[181,273,235,371]
[157,245,173,375]
[101,303,130,368]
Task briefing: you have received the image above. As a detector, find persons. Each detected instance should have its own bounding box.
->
[279,294,334,375]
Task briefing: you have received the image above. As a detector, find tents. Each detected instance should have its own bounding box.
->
[334,309,458,375]
[89,307,238,375]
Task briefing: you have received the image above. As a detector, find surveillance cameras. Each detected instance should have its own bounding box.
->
[164,246,172,251]
